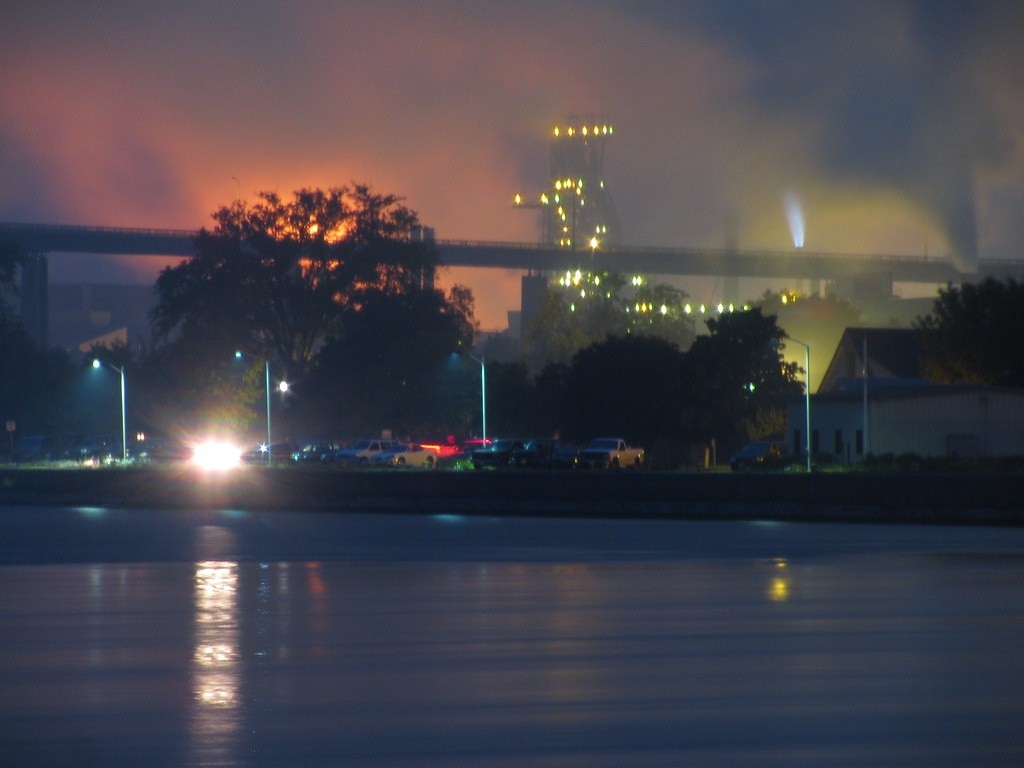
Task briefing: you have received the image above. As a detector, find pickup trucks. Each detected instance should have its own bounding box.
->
[729,440,801,472]
[578,435,646,472]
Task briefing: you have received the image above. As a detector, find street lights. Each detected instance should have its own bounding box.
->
[92,358,127,462]
[234,349,272,469]
[782,332,812,473]
[457,338,487,449]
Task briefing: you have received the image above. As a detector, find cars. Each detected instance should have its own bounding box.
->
[8,433,170,463]
[245,430,583,469]
